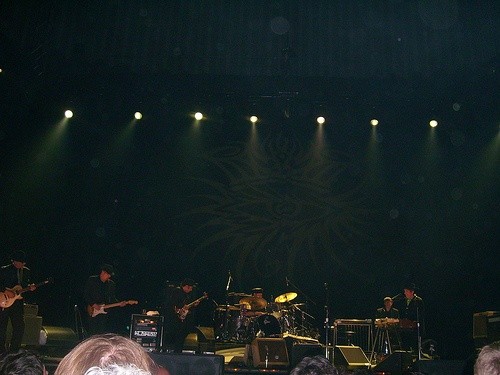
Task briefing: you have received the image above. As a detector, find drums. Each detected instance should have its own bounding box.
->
[272,309,292,327]
[215,294,231,311]
[254,312,291,338]
[242,315,259,326]
[246,316,262,344]
[226,296,243,311]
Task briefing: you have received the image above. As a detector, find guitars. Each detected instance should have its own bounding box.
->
[0,280,49,308]
[87,300,138,318]
[174,295,208,320]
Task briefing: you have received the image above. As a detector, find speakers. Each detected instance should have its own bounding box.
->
[333,324,373,371]
[473,311,500,339]
[4,315,77,348]
[372,351,413,375]
[150,350,225,375]
[182,326,215,351]
[251,337,323,370]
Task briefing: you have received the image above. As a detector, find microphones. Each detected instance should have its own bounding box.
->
[285,276,288,286]
[229,272,234,282]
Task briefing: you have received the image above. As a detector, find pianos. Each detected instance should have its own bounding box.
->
[374,318,417,360]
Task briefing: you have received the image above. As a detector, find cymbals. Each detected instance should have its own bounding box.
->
[246,295,268,310]
[286,303,306,312]
[239,297,251,310]
[275,292,298,303]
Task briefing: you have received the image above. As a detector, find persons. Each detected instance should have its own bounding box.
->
[85,264,127,336]
[54,333,170,375]
[252,288,263,297]
[171,279,206,354]
[474,341,500,375]
[290,355,337,375]
[376,297,398,353]
[0,250,36,354]
[398,278,425,351]
[0,348,48,375]
